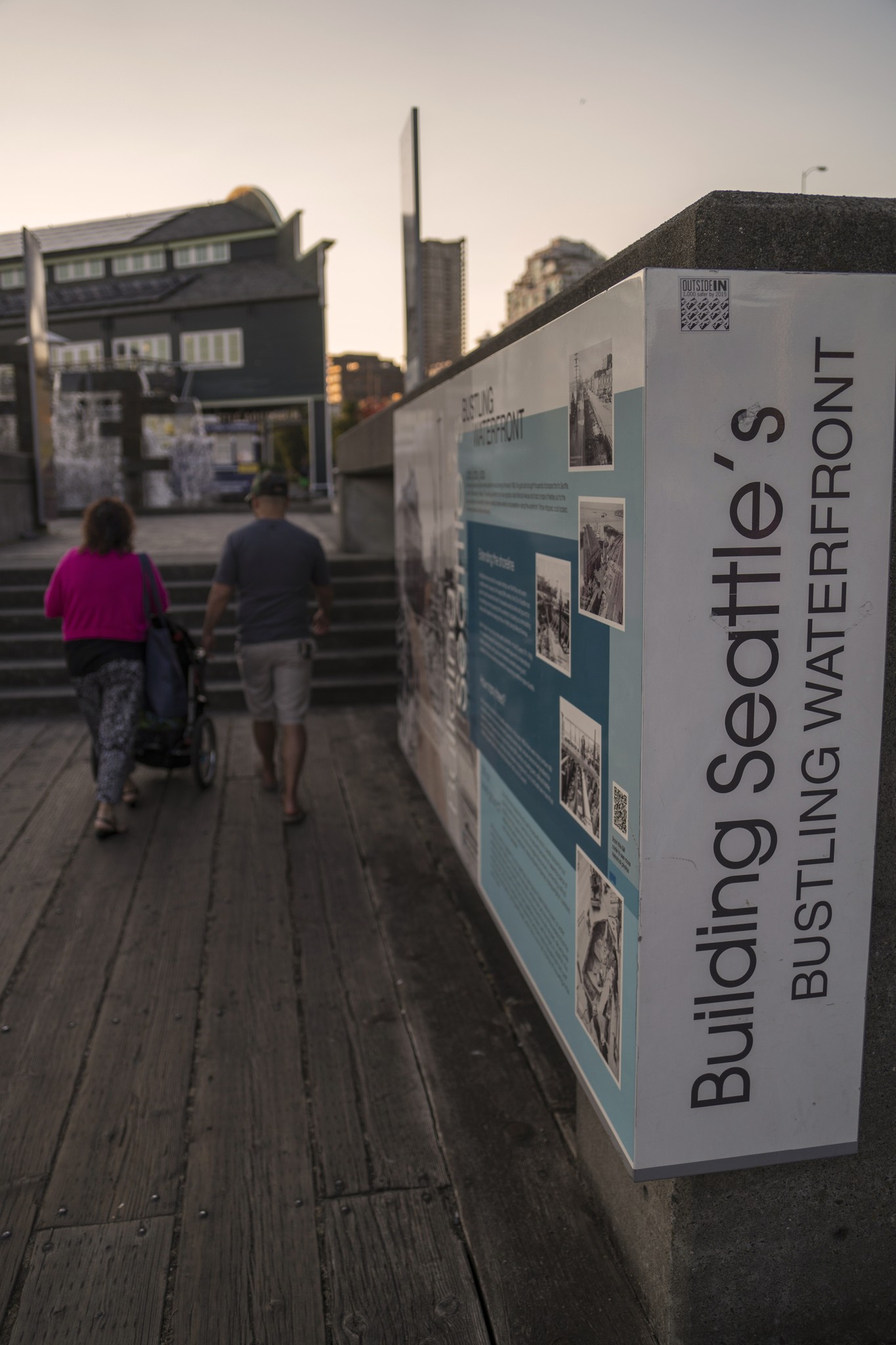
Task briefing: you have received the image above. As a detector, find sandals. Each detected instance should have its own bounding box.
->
[95,814,128,839]
[123,787,140,806]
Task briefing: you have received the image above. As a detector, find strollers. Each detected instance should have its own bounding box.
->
[84,615,225,785]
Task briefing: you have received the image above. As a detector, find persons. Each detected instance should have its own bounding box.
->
[46,498,170,841]
[200,469,334,825]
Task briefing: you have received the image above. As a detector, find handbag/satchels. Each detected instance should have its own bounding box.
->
[140,552,188,717]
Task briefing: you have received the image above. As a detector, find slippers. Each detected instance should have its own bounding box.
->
[263,775,278,791]
[282,802,304,824]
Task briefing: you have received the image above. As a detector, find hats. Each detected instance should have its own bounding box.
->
[244,469,288,502]
